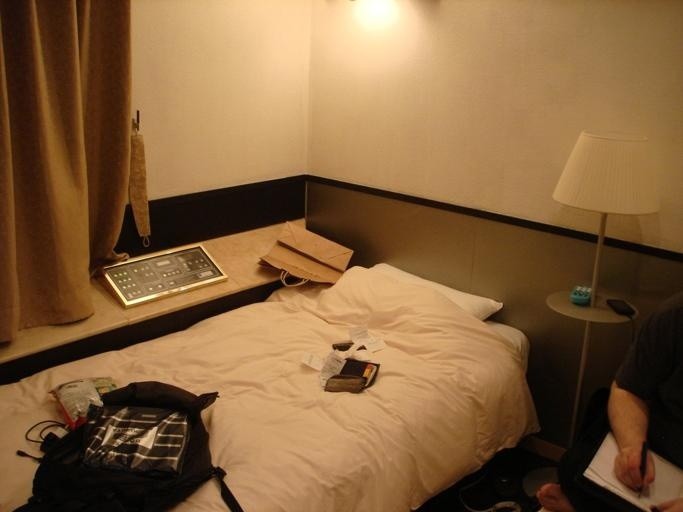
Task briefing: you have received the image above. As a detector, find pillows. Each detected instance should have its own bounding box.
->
[370,263,504,322]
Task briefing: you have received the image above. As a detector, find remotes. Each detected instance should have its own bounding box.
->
[570,284,592,304]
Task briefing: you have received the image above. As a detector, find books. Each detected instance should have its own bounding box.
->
[581,429,683,512]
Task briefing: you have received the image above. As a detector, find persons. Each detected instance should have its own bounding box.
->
[532,279,683,512]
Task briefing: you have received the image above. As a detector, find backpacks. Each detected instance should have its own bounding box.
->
[17,380,225,512]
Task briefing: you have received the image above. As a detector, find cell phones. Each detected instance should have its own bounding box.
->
[607,299,634,315]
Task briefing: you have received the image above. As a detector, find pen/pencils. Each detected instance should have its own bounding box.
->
[638,442,648,498]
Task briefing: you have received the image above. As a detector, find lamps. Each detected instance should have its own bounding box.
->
[522,130,658,497]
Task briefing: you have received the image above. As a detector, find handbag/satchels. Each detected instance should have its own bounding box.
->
[255,220,354,290]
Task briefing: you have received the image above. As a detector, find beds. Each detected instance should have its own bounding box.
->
[0,280,539,512]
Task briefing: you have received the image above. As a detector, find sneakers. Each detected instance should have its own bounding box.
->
[457,470,526,512]
